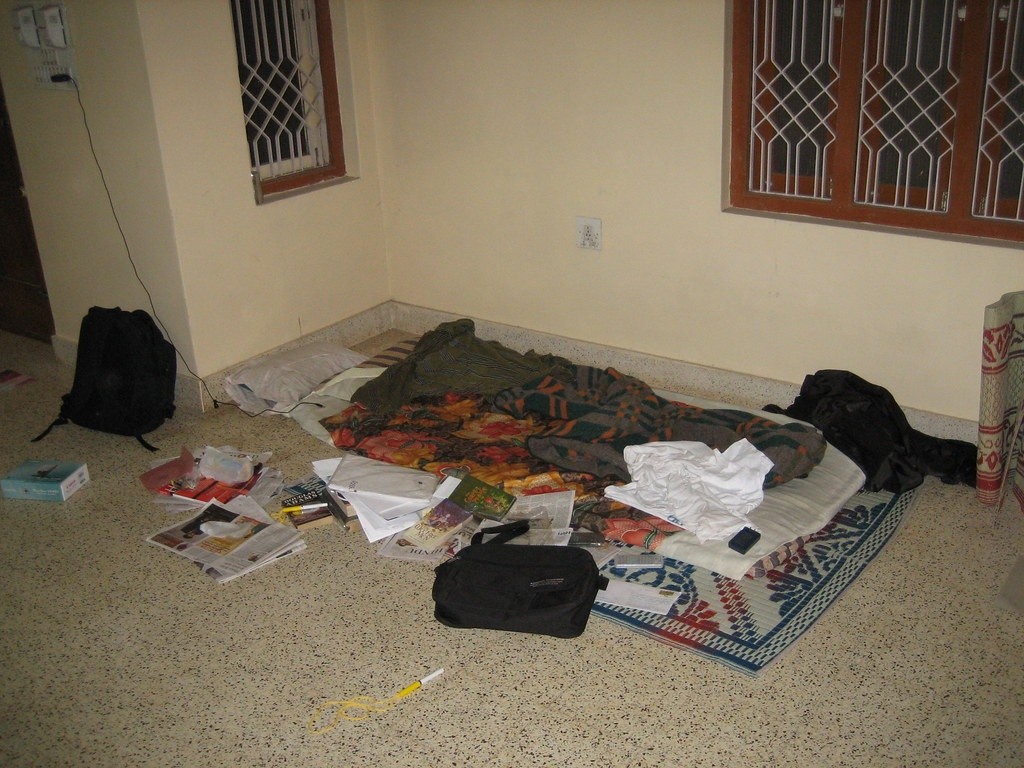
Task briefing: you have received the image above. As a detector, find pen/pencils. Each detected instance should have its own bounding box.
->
[398,668,444,697]
[283,503,327,512]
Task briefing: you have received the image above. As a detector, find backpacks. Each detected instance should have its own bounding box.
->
[31,306,177,453]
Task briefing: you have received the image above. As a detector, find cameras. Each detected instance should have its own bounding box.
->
[729,526,761,555]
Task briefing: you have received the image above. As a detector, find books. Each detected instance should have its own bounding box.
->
[614,554,664,569]
[282,474,517,550]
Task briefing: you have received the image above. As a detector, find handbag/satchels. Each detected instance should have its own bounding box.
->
[432,520,609,639]
